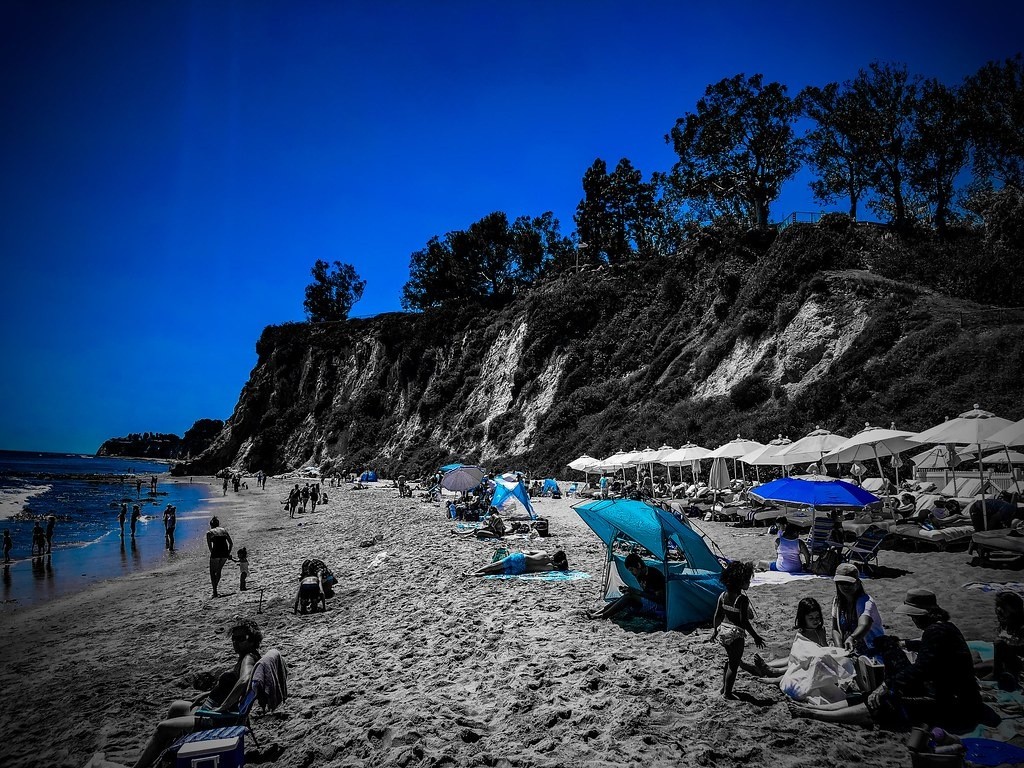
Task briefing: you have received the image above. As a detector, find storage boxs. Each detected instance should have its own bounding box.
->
[176,731,245,768]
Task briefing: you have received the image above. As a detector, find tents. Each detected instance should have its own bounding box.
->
[543,480,558,497]
[491,482,537,519]
[569,497,759,631]
[361,470,378,481]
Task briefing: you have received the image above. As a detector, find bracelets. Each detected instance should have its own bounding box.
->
[850,634,856,641]
[881,685,885,691]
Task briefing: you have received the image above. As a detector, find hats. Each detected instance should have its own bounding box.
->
[834,563,860,583]
[893,589,944,615]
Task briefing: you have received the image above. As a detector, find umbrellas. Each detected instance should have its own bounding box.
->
[440,463,484,496]
[769,402,1024,496]
[748,470,882,568]
[567,434,794,484]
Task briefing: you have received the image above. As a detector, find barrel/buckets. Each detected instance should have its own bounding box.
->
[907,726,932,751]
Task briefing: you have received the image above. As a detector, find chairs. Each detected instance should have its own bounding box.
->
[194,650,287,750]
[564,476,1024,576]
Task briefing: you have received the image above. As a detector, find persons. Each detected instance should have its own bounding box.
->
[445,481,490,522]
[206,515,248,599]
[517,470,542,497]
[450,506,568,576]
[586,553,668,619]
[393,471,444,502]
[116,467,177,553]
[1,516,55,564]
[190,472,328,519]
[590,466,686,499]
[551,486,555,492]
[757,493,1024,571]
[330,470,365,490]
[133,620,264,768]
[711,517,1024,720]
[293,558,334,614]
